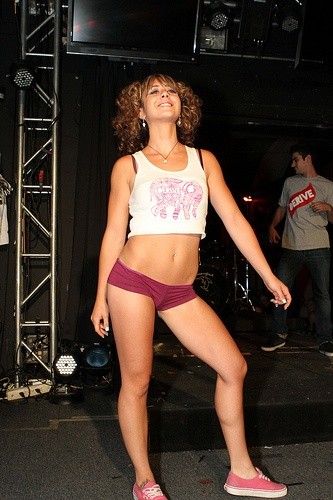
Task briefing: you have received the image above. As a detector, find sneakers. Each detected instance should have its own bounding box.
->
[318,342,333,357]
[261,337,286,351]
[133,481,167,500]
[224,466,287,498]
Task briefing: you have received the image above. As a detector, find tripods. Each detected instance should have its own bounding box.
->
[224,248,257,313]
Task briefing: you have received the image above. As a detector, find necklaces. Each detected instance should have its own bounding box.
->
[147,141,179,164]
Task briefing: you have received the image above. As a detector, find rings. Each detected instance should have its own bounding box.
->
[270,240,272,243]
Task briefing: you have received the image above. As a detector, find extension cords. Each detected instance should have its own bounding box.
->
[6,382,52,400]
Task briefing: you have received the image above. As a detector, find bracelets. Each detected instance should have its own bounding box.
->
[326,207,333,214]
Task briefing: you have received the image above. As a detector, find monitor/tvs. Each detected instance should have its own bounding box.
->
[66,0,203,64]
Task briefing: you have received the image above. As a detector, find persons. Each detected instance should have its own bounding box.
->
[255,294,264,312]
[260,143,333,356]
[91,74,291,500]
